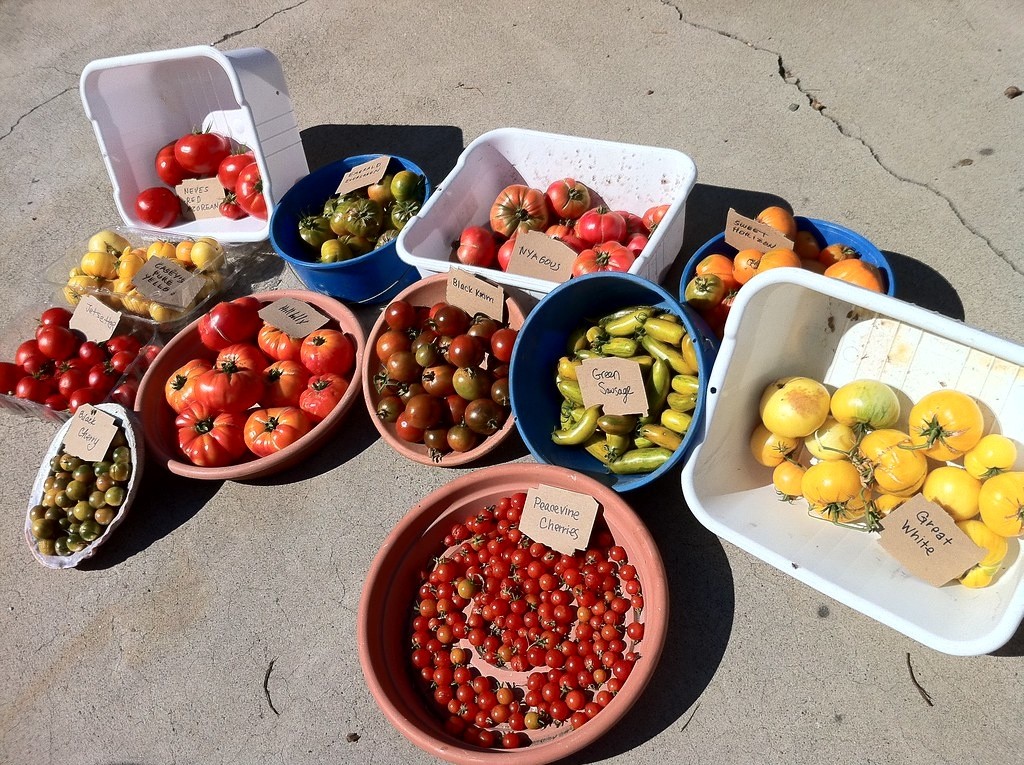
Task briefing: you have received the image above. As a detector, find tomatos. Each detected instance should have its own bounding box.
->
[0,122,1024,748]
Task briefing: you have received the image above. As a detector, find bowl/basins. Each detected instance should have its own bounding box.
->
[677,217,896,302]
[40,226,228,331]
[0,303,165,432]
[361,271,527,467]
[269,152,429,309]
[133,288,368,482]
[508,271,720,492]
[24,401,146,570]
[356,462,671,764]
